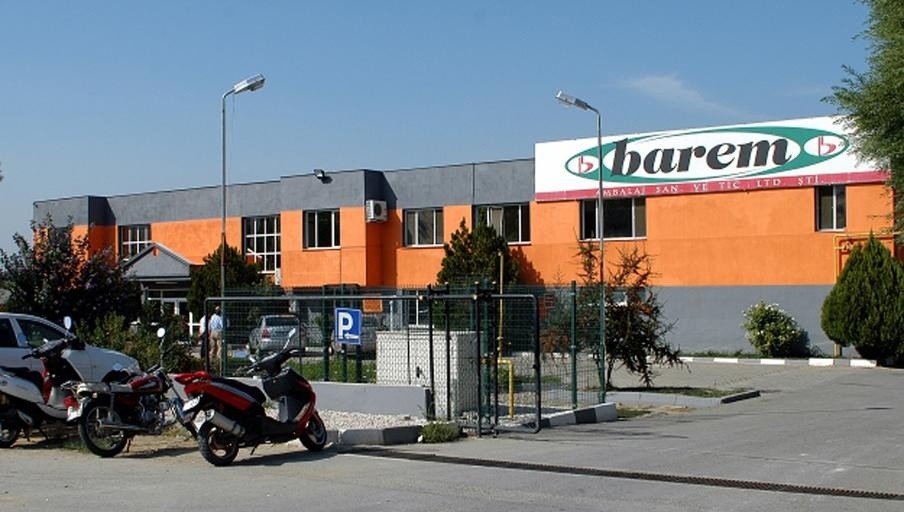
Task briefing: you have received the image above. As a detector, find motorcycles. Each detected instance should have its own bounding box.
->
[0,316,88,449]
[174,327,327,467]
[60,327,198,458]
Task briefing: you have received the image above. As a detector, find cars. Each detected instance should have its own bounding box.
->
[0,311,141,384]
[248,314,307,357]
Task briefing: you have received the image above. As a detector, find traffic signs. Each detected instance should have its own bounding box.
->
[335,307,362,345]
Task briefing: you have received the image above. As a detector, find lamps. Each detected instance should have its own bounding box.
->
[313,168,327,182]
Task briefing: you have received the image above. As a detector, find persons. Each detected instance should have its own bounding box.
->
[198,310,210,359]
[207,306,223,358]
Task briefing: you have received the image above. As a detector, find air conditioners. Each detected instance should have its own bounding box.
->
[364,199,387,224]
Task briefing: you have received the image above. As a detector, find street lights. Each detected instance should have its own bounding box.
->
[218,72,266,379]
[554,88,608,404]
